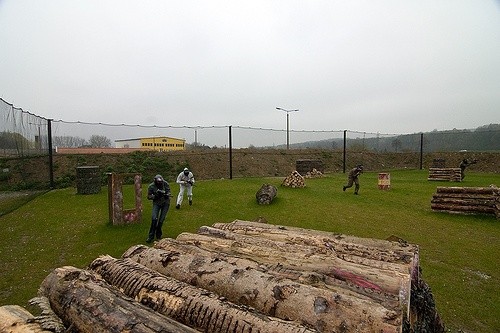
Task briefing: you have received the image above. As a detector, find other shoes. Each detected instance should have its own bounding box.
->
[176,205,180,209]
[354,192,358,195]
[189,200,192,205]
[146,238,151,242]
[343,186,346,191]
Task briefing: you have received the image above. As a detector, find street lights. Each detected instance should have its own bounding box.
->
[276,107,299,149]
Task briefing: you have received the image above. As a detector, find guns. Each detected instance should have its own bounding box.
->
[355,170,364,174]
[147,190,174,200]
[181,178,198,187]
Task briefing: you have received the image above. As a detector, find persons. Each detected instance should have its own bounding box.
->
[460,159,473,181]
[175,167,194,208]
[146,174,170,243]
[343,165,363,195]
